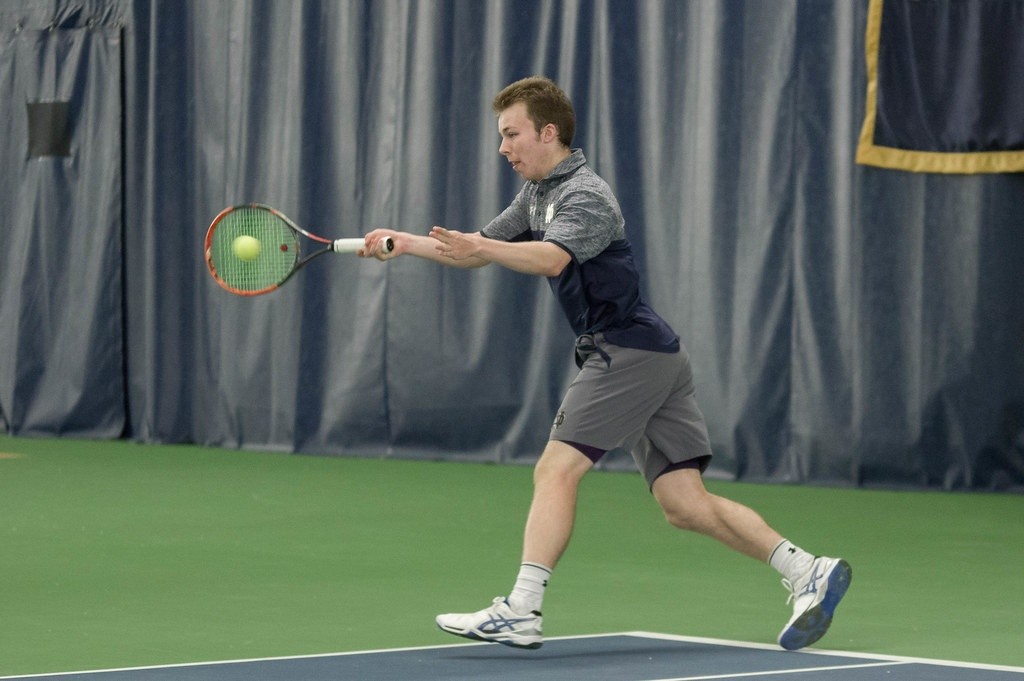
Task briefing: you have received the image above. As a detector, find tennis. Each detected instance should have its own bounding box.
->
[232,235,260,261]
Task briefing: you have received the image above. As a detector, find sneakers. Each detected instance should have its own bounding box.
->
[777,556,854,652]
[435,597,555,648]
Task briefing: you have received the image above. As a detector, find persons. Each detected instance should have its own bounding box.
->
[358,75,852,650]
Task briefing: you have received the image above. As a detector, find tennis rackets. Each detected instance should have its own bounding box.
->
[204,202,395,298]
